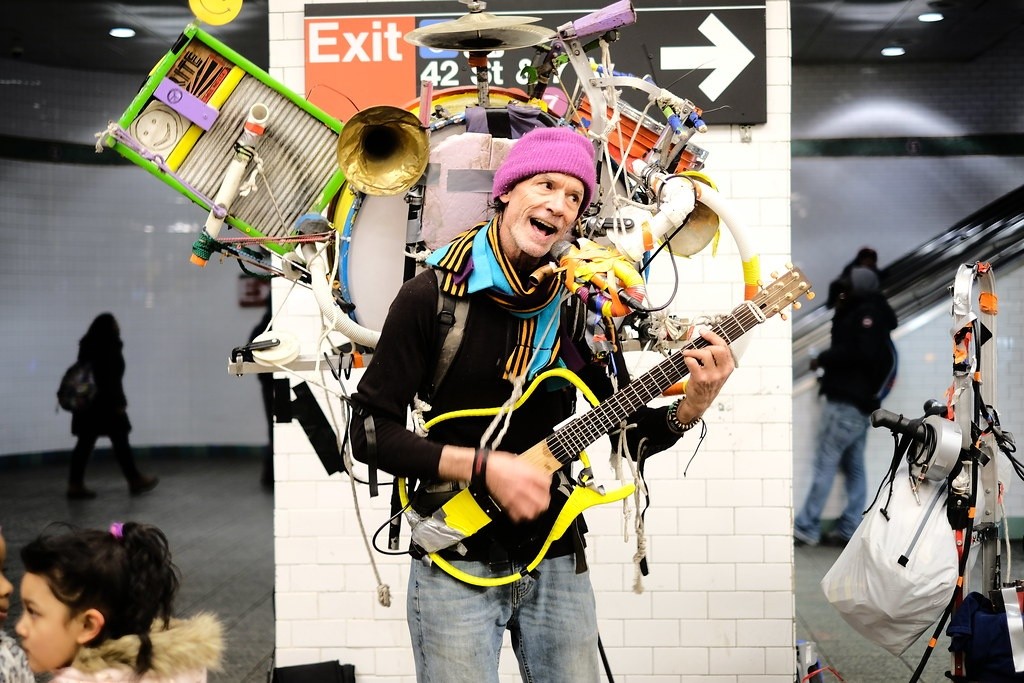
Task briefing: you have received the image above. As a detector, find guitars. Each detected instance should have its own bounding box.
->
[397,263,815,587]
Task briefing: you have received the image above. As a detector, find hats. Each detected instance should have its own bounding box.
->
[492,126,597,219]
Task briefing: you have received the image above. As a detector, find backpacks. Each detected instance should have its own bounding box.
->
[58,360,102,412]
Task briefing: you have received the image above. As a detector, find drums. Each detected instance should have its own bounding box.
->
[328,85,650,351]
[570,88,710,183]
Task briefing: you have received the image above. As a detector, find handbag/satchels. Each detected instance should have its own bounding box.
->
[820,462,961,658]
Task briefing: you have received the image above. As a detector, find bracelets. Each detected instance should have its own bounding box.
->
[672,396,707,477]
[470,447,488,491]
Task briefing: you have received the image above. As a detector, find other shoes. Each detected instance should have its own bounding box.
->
[128,475,160,497]
[821,529,850,548]
[67,485,97,500]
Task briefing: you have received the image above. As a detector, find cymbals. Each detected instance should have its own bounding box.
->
[403,20,559,52]
[414,12,543,34]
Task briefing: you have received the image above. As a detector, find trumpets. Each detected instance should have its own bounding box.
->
[337,106,430,196]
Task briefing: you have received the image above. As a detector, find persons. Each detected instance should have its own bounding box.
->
[794,246,897,548]
[57,311,160,503]
[0,524,35,683]
[14,521,226,683]
[350,127,735,683]
[247,303,274,495]
